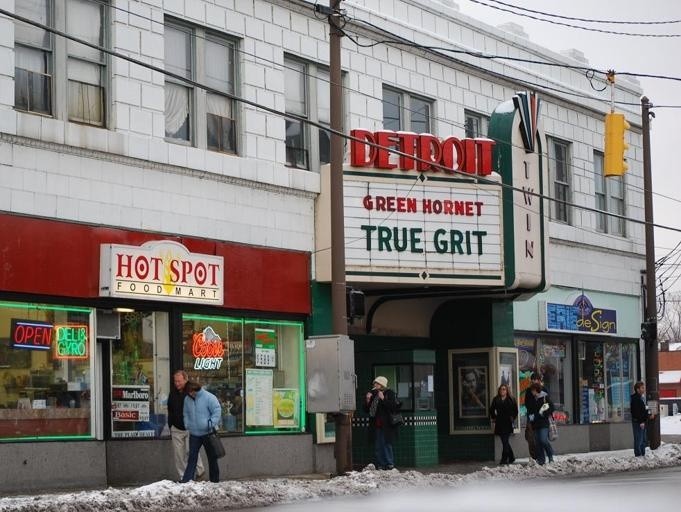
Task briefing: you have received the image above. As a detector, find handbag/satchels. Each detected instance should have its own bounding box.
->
[384,420,400,441]
[538,403,550,417]
[205,418,226,460]
[548,415,559,441]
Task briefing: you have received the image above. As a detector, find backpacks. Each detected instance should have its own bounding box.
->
[371,388,403,417]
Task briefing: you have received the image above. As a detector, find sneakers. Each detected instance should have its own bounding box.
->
[376,464,395,470]
[497,457,516,466]
[196,471,207,482]
[533,456,555,465]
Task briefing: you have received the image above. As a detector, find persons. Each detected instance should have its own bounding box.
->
[174,379,222,482]
[167,369,206,483]
[631,380,655,457]
[132,363,149,386]
[462,369,487,415]
[531,383,555,465]
[525,372,548,466]
[363,376,396,471]
[488,383,519,465]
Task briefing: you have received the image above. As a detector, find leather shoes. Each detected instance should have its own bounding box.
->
[179,479,195,483]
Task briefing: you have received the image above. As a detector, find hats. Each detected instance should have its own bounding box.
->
[374,375,389,388]
